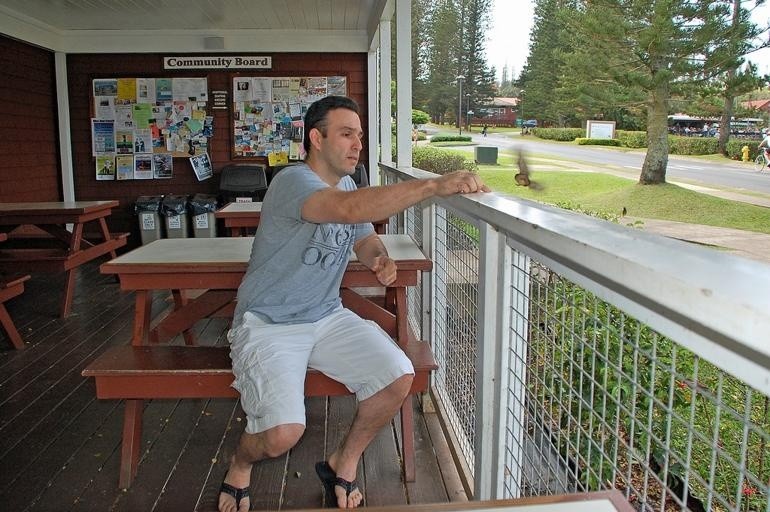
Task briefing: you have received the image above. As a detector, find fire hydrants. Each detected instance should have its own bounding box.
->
[742,146,750,163]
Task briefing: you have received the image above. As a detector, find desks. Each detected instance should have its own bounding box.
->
[98,235,433,341]
[0,201,131,317]
[213,202,390,236]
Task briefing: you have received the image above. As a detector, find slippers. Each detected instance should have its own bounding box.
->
[315,461,364,508]
[217,470,253,512]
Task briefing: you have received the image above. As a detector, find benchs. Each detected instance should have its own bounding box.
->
[81,340,440,491]
[0,274,32,351]
[166,286,396,308]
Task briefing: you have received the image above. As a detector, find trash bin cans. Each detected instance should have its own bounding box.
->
[134,164,299,246]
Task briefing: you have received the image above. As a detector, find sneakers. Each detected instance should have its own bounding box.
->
[767,162,770,167]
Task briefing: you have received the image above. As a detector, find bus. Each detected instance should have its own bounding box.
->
[668,115,764,130]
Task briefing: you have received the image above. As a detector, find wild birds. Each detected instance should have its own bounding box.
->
[622,207,627,217]
[513,151,545,191]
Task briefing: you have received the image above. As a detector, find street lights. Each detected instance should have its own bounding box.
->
[456,74,474,135]
[519,90,526,116]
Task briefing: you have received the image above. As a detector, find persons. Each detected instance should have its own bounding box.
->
[218,96,492,512]
[757,132,770,167]
[703,122,711,137]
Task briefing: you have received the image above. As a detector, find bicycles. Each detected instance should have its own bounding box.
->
[754,146,770,172]
[481,129,488,140]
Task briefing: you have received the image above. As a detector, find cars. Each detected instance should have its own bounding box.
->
[517,119,538,128]
